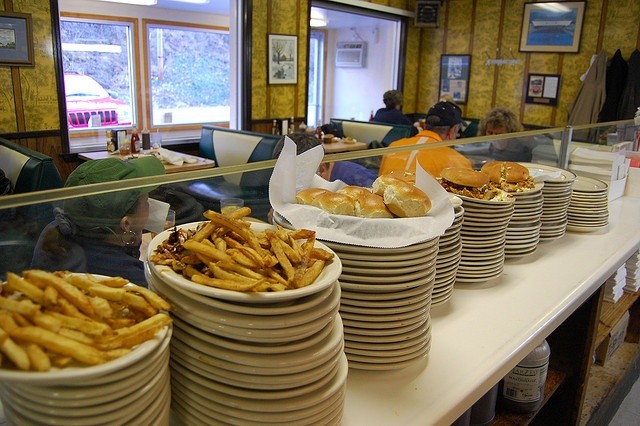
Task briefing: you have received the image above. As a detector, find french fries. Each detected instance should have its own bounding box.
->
[0,269,173,372]
[154,206,257,300]
[258,227,334,293]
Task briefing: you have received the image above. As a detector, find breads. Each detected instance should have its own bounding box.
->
[384,184,431,217]
[313,193,356,217]
[372,176,406,196]
[296,188,330,206]
[337,185,371,201]
[355,193,391,217]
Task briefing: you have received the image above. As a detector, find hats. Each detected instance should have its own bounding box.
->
[63,156,167,228]
[425,101,462,127]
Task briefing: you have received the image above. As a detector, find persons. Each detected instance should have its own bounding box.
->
[374,90,418,137]
[268,131,382,227]
[29,155,166,290]
[478,109,536,162]
[439,95,452,102]
[377,102,474,176]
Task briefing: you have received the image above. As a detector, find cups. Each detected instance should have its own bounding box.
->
[221,198,244,215]
[150,209,175,238]
[117,131,125,152]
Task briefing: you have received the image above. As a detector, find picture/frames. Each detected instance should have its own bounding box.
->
[518,1,587,54]
[437,54,471,105]
[1,11,36,69]
[267,33,299,84]
[524,73,561,106]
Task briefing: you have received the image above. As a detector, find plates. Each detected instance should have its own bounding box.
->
[1,273,173,426]
[505,179,545,261]
[566,177,609,232]
[430,206,465,308]
[465,155,490,165]
[517,162,578,242]
[436,177,516,283]
[271,205,440,371]
[144,220,348,426]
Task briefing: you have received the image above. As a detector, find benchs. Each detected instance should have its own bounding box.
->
[183,127,280,203]
[413,114,478,137]
[329,119,415,149]
[0,136,40,205]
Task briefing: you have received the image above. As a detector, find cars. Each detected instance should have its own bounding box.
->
[63,73,131,129]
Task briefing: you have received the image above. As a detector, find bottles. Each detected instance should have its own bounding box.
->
[131,125,140,152]
[272,120,276,135]
[119,139,130,156]
[289,117,295,132]
[502,339,551,413]
[369,111,374,120]
[275,122,281,136]
[141,126,151,149]
[315,126,324,144]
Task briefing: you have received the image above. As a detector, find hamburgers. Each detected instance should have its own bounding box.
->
[439,168,506,201]
[481,161,535,190]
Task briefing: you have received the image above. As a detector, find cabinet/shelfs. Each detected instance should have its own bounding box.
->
[137,139,637,422]
